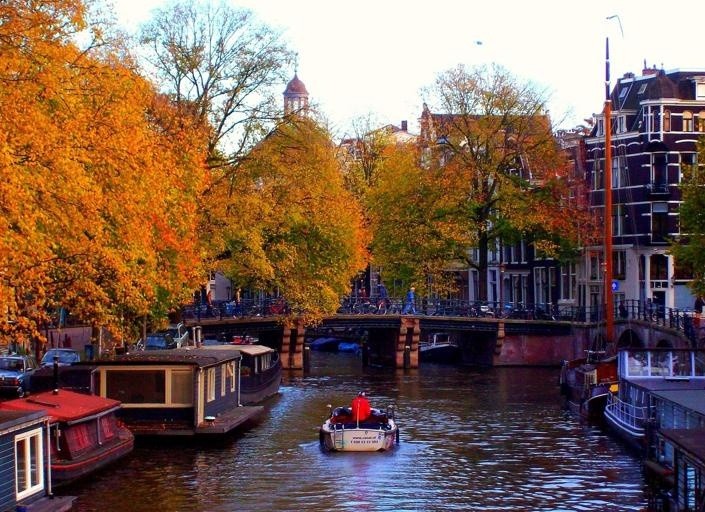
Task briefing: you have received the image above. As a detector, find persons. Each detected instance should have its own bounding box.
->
[695,295,705,313]
[206,290,213,305]
[400,286,419,316]
[351,390,372,422]
[360,287,372,304]
[234,288,242,304]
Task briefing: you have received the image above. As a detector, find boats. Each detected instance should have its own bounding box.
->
[655,429,705,512]
[31,347,265,444]
[0,409,81,512]
[0,357,147,493]
[605,350,705,450]
[181,327,282,407]
[640,390,705,483]
[205,328,363,356]
[559,348,675,420]
[419,331,460,364]
[319,392,402,454]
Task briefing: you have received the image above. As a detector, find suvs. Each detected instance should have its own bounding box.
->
[156,323,191,350]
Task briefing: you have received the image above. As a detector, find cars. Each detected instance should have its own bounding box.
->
[0,355,39,396]
[40,348,82,370]
[146,332,176,350]
